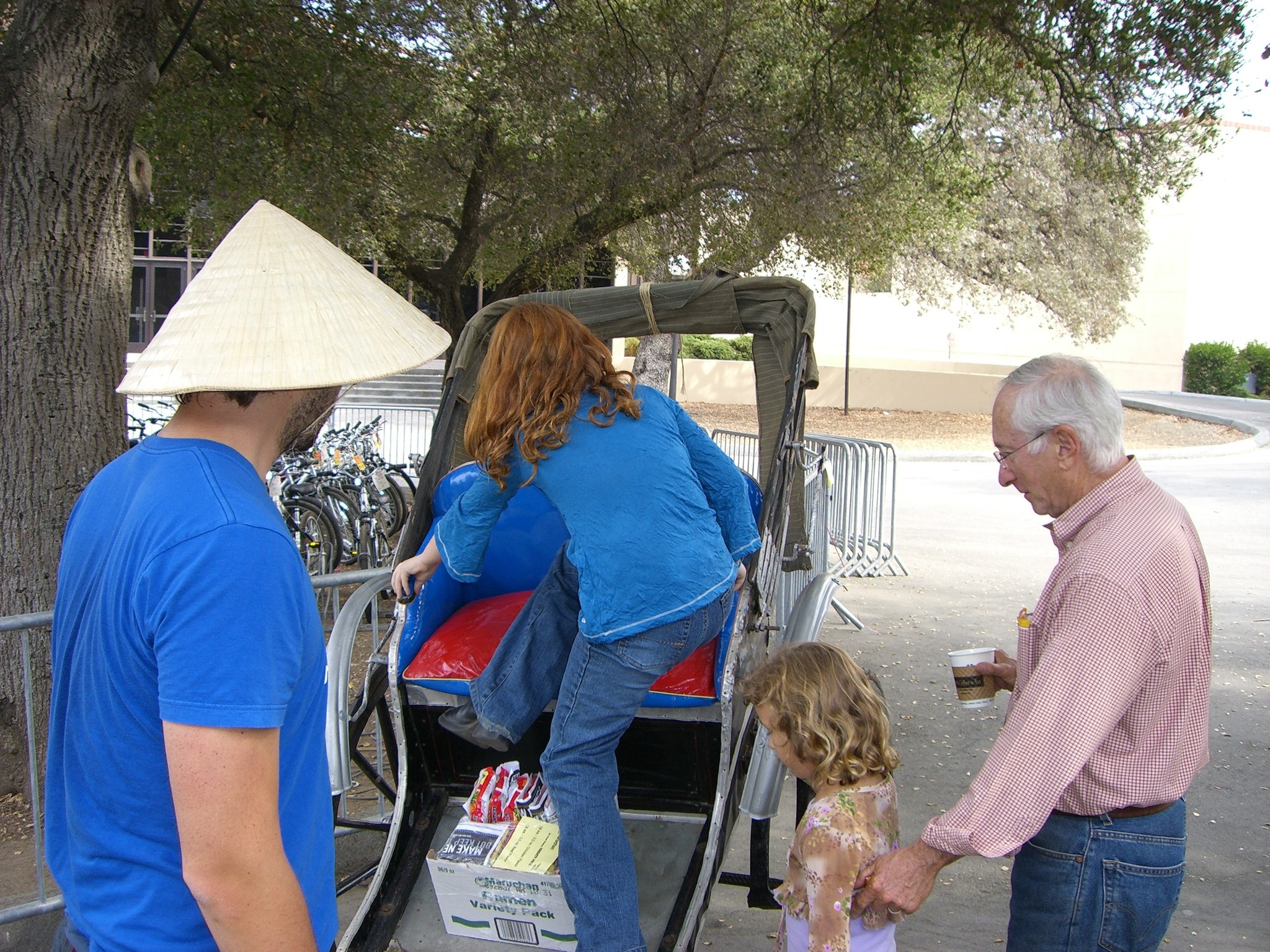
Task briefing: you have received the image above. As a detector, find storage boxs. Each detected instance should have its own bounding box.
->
[426,849,578,952]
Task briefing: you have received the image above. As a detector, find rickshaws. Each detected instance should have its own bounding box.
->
[328,267,834,952]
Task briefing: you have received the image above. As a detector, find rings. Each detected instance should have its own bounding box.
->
[887,907,903,914]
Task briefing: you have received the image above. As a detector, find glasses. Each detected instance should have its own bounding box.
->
[993,427,1053,469]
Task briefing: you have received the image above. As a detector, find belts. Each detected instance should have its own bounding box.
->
[1050,801,1173,818]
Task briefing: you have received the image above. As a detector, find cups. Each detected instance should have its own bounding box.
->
[947,647,996,709]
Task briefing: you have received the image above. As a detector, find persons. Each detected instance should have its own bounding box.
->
[844,354,1216,952]
[41,199,454,950]
[737,639,904,952]
[391,304,756,952]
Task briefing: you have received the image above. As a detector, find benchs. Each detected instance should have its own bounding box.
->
[397,460,766,723]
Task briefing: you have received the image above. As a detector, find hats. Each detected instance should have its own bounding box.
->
[115,200,453,396]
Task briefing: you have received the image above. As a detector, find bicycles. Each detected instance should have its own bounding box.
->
[125,401,424,624]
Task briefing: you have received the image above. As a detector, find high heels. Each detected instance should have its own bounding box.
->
[437,702,510,752]
